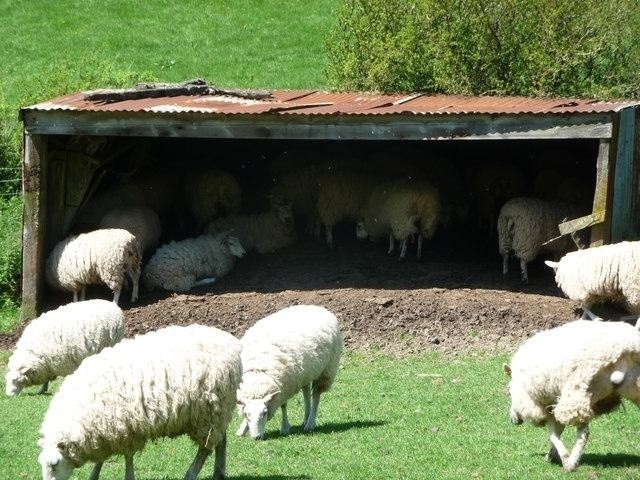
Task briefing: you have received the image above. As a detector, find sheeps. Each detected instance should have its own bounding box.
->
[43,228,142,305]
[267,169,441,261]
[543,240,639,322]
[234,303,344,440]
[100,168,298,253]
[497,195,593,285]
[37,323,241,480]
[4,299,126,398]
[143,233,244,291]
[503,321,640,473]
